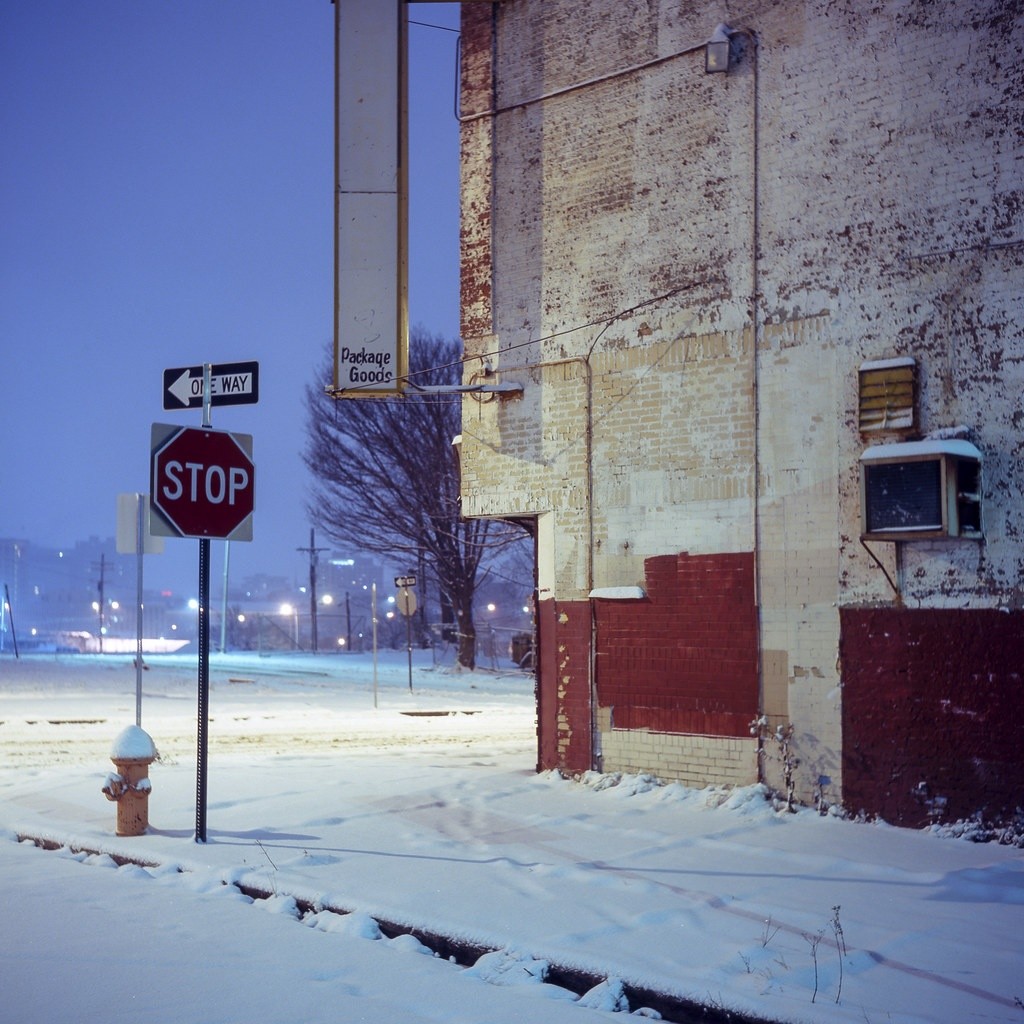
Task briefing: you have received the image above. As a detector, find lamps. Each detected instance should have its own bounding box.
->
[706,25,734,74]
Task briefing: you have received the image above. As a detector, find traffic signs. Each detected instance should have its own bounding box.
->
[164,362,260,411]
[394,575,416,588]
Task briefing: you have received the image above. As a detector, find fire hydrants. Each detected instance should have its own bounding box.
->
[101,724,158,836]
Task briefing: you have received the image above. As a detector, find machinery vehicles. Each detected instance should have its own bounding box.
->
[3,638,56,654]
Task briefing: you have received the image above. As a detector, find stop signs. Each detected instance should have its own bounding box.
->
[149,421,256,540]
